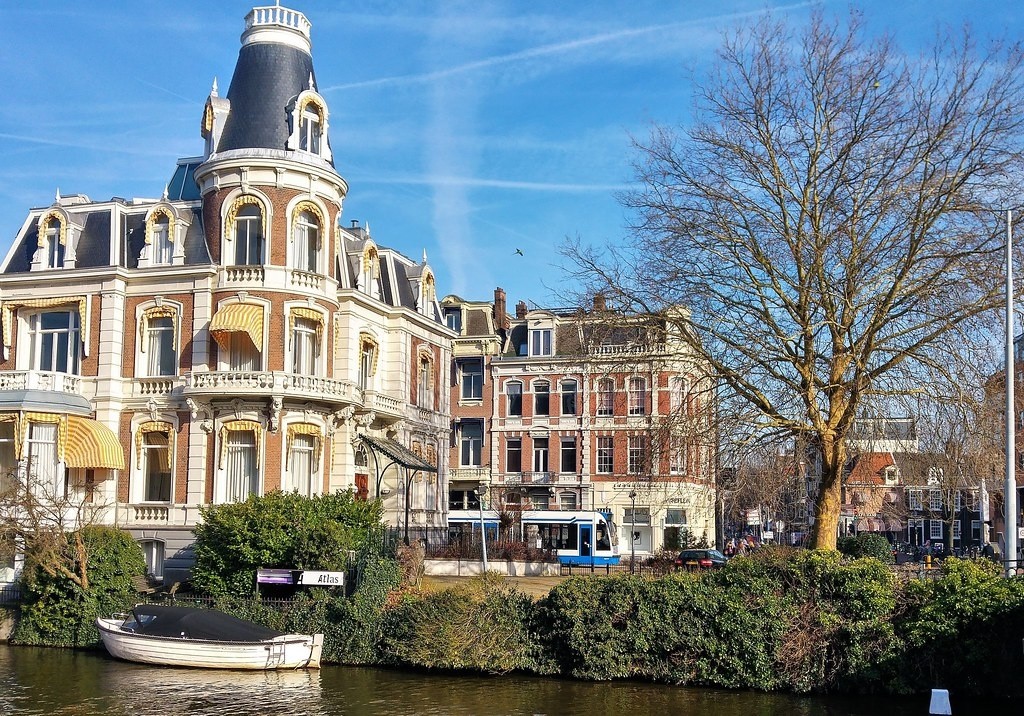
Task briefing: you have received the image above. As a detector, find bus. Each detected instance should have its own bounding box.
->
[446,509,621,566]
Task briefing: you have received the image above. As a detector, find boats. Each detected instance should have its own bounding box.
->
[94,603,324,670]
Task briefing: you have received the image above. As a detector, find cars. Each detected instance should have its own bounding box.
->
[674,548,728,574]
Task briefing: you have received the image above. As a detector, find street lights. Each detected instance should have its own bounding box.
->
[628,492,637,572]
[472,485,489,572]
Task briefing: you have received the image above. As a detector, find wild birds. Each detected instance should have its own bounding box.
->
[513,248,523,256]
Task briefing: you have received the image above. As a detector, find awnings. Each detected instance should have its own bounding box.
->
[858,517,902,531]
[854,490,869,502]
[148,432,170,473]
[64,417,124,470]
[885,493,902,503]
[209,305,262,351]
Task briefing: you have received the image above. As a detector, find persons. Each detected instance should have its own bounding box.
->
[891,544,898,564]
[982,541,994,560]
[923,540,931,555]
[723,534,762,558]
